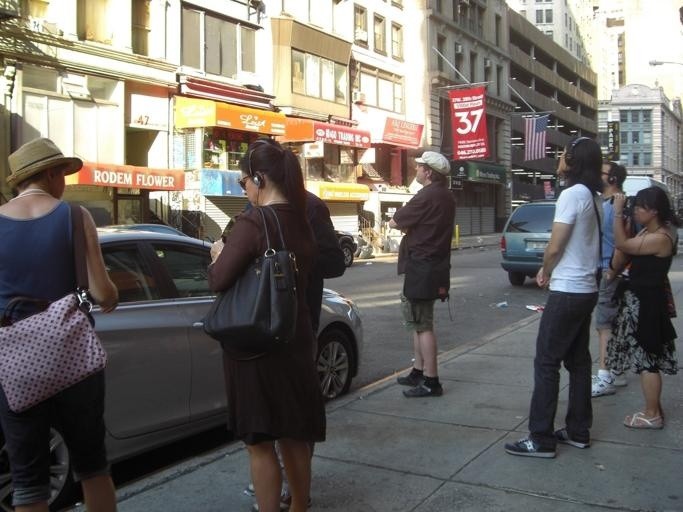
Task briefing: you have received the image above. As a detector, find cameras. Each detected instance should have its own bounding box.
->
[610,195,636,216]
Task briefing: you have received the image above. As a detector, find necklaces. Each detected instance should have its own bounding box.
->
[265,199,288,205]
[18,189,46,196]
[654,224,663,233]
[9,192,53,201]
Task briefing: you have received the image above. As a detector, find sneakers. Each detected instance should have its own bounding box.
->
[505,436,556,458]
[396,373,420,384]
[592,375,616,396]
[554,426,591,449]
[404,379,442,398]
[611,370,627,386]
[242,463,313,510]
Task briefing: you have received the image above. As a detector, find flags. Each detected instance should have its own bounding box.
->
[524,115,548,162]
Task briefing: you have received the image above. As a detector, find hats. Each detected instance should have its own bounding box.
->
[4,137,83,188]
[415,151,451,175]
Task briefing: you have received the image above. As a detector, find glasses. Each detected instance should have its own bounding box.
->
[237,176,253,190]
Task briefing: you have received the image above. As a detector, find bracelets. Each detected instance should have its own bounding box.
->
[614,213,623,217]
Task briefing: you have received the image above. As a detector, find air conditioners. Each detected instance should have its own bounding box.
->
[355,29,368,42]
[353,91,367,103]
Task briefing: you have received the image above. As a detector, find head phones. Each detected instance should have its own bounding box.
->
[608,162,617,184]
[248,148,265,188]
[565,136,591,166]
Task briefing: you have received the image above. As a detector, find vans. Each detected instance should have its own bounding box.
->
[623,176,682,255]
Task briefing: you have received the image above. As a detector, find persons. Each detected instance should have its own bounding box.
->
[244,138,346,512]
[613,186,678,430]
[389,151,455,396]
[503,136,603,458]
[207,145,320,512]
[0,138,119,512]
[591,161,628,398]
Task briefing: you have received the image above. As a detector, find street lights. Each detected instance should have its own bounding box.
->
[665,171,682,182]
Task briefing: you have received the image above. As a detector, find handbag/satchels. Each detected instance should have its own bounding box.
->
[202,206,299,344]
[1,284,108,412]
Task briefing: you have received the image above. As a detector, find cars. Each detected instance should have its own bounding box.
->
[333,230,358,267]
[103,224,207,278]
[500,200,556,286]
[1,229,364,511]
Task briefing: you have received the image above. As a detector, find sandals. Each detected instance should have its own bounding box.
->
[624,412,665,430]
